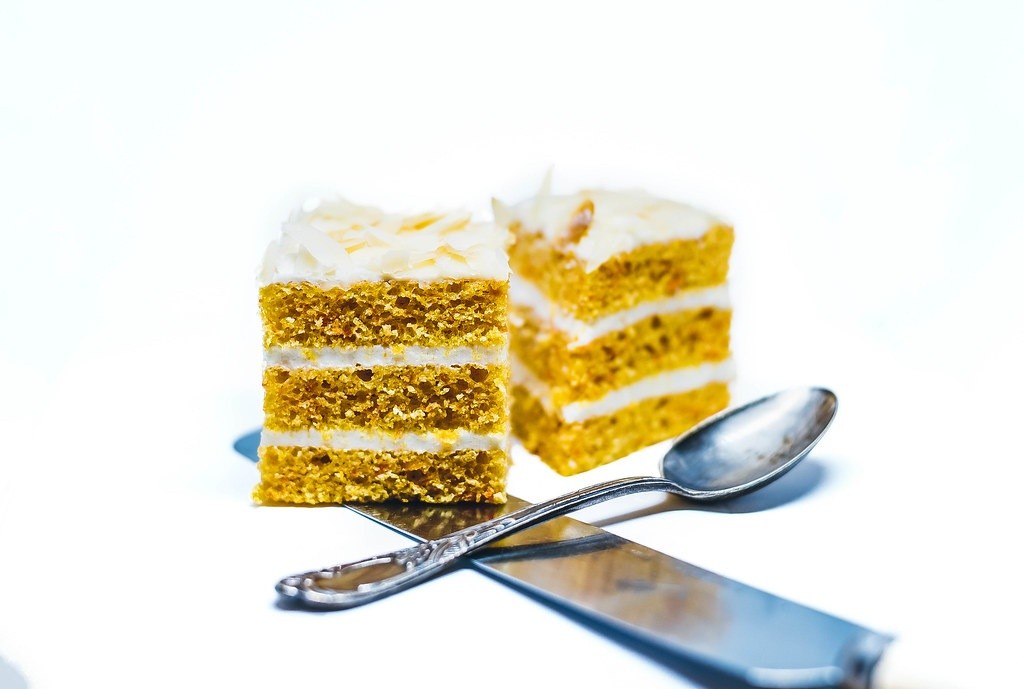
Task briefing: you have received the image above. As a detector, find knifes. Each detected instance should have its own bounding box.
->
[233,431,907,689]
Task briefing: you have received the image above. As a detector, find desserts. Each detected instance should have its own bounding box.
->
[490,187,736,476]
[251,193,519,507]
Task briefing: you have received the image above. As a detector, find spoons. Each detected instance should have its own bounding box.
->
[274,388,836,610]
